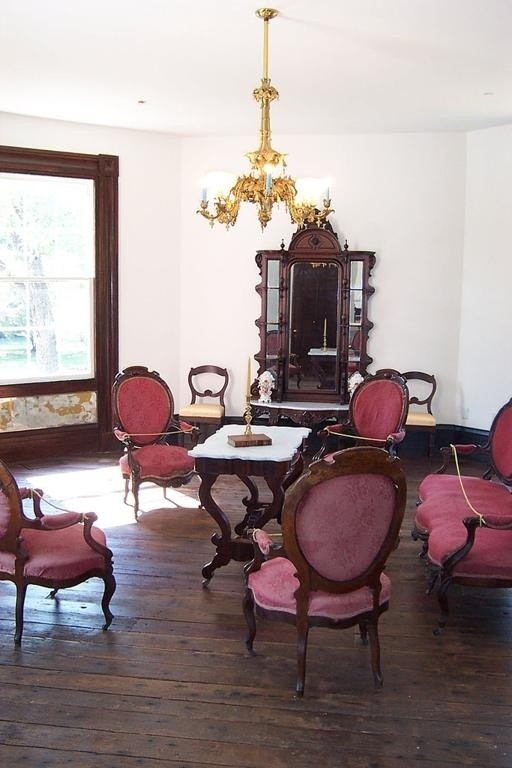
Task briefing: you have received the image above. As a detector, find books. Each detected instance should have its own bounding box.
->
[227,434,272,447]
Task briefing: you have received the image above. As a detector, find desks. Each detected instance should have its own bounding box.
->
[186,423,311,588]
[243,399,351,460]
[307,344,336,390]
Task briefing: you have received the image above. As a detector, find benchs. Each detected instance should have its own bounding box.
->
[412,396,511,635]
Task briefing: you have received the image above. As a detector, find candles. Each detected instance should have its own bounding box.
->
[245,358,251,398]
[322,315,328,336]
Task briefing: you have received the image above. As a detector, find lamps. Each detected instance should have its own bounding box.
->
[195,10,335,235]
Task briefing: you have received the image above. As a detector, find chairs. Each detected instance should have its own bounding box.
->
[344,327,361,382]
[109,366,204,523]
[312,367,409,471]
[179,365,229,447]
[1,457,118,650]
[399,370,437,468]
[241,446,406,700]
[266,328,305,391]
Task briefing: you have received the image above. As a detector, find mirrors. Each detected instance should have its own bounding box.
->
[246,215,377,406]
